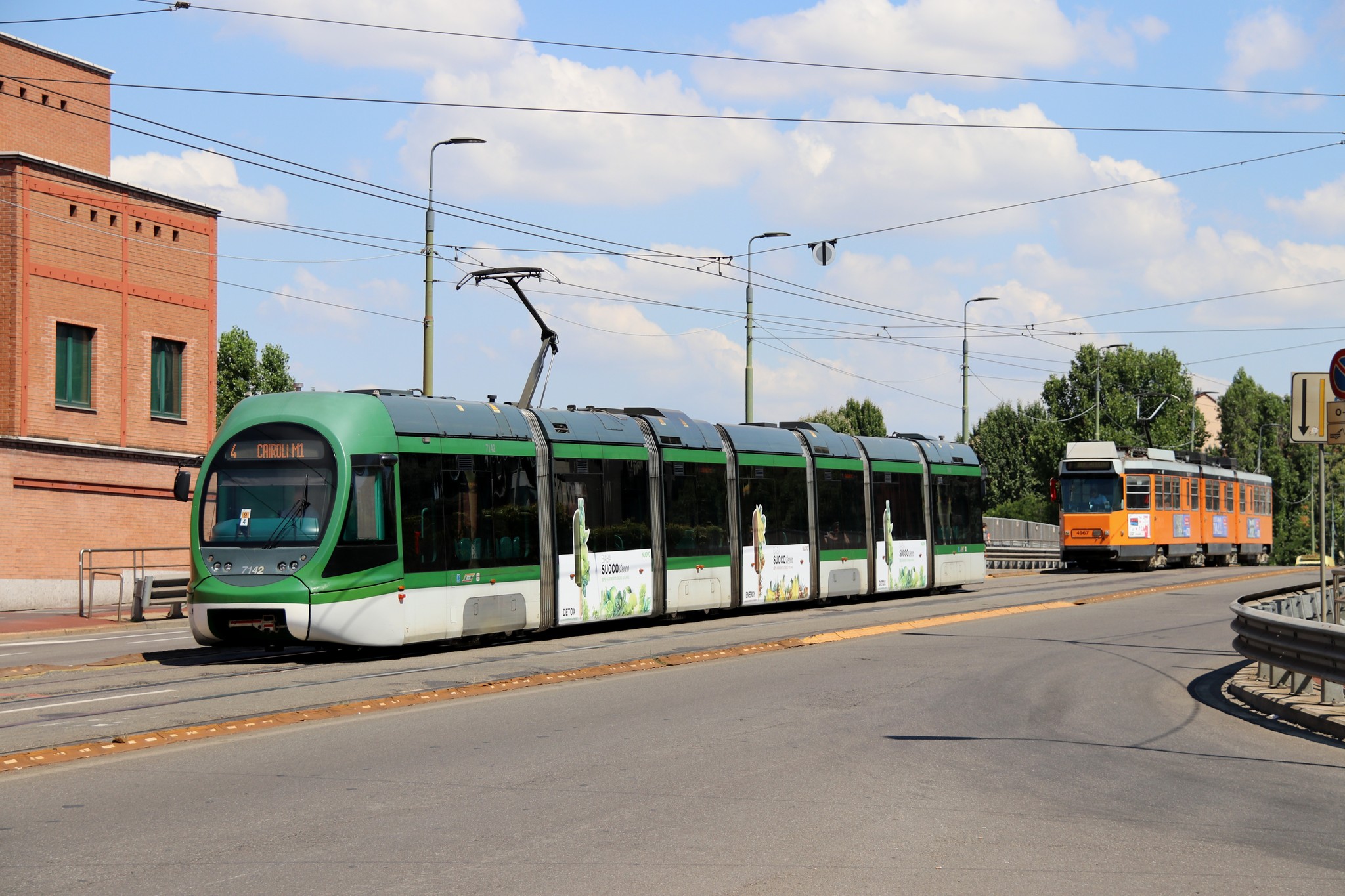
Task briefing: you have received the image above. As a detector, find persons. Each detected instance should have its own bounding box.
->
[823,521,851,544]
[983,522,991,564]
[1087,488,1107,504]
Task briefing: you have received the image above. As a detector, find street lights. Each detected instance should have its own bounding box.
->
[1330,483,1345,558]
[1257,423,1284,474]
[962,296,1000,445]
[1097,344,1128,443]
[423,137,489,398]
[1190,391,1220,452]
[746,232,792,423]
[1312,452,1332,555]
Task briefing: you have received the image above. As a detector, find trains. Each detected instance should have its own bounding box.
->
[173,388,986,656]
[1050,392,1273,572]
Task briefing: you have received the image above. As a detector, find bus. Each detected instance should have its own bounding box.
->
[1295,554,1335,567]
